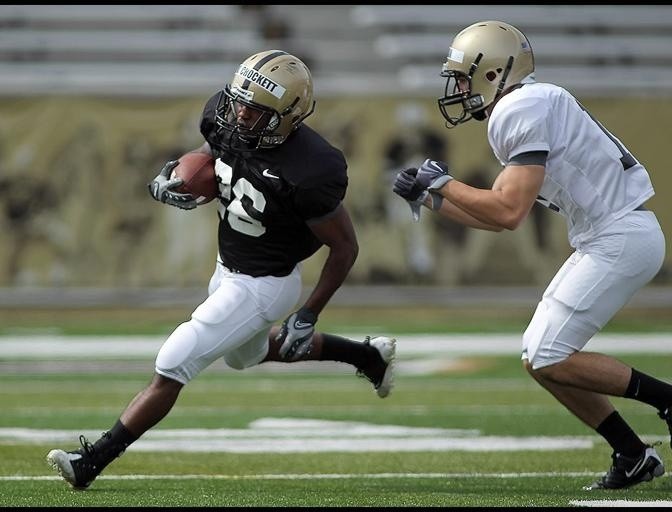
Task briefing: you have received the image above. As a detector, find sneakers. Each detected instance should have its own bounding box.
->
[45,439,114,492]
[587,448,661,498]
[355,333,402,400]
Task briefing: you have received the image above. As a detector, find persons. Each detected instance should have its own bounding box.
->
[381,103,449,279]
[46,47,395,489]
[394,19,671,492]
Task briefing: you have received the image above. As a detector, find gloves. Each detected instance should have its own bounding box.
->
[415,157,450,189]
[146,160,197,213]
[276,311,316,363]
[393,170,425,203]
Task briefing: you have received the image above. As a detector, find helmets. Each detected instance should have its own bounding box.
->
[215,48,312,146]
[437,18,537,123]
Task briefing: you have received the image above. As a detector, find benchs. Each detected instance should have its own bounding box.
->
[0,2,670,99]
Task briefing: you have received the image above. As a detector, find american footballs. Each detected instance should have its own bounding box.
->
[170,152,219,205]
